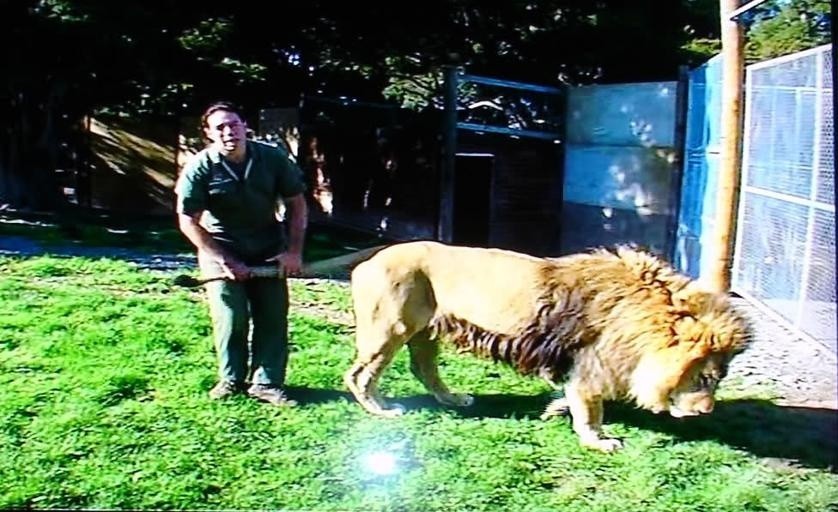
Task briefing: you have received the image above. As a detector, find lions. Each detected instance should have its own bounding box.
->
[172,239,755,451]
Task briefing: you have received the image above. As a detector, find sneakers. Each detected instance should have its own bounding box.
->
[208,380,240,399]
[247,383,299,409]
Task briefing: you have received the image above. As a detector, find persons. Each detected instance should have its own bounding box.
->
[172,100,309,410]
